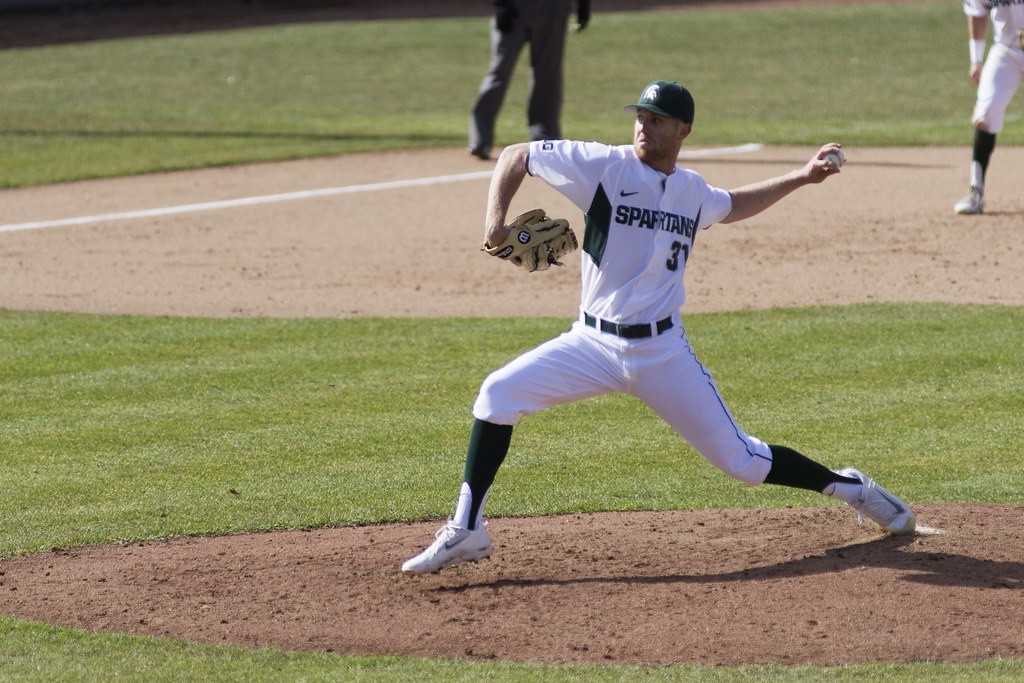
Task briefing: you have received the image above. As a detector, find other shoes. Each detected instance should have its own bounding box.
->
[470,142,489,159]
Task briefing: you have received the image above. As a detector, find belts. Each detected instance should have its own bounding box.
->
[585,314,674,339]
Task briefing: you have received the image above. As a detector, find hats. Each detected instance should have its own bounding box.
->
[623,80,694,124]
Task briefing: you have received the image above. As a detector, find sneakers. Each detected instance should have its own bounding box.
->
[402,515,492,579]
[955,186,984,214]
[842,468,916,535]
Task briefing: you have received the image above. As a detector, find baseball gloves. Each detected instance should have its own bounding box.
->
[477,206,579,274]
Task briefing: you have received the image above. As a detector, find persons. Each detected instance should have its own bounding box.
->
[469,0,592,158]
[955,0,1024,215]
[400,80,917,576]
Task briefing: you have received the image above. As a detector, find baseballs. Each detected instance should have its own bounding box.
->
[823,144,846,171]
